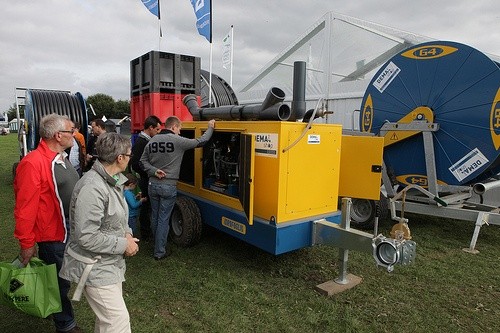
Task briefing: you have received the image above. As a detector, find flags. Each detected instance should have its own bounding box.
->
[139,0,158,19]
[221,30,232,68]
[189,0,210,43]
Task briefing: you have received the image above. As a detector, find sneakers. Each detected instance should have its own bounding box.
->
[56,324,85,333]
[154,250,178,262]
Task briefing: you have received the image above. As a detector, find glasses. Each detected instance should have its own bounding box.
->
[115,152,133,160]
[54,129,74,137]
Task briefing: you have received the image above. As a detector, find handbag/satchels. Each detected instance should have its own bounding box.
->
[0,254,62,318]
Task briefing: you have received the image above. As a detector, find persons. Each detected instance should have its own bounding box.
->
[58,132,139,333]
[62,118,116,179]
[139,116,216,261]
[13,112,85,333]
[1,126,8,135]
[124,174,147,238]
[129,116,162,240]
